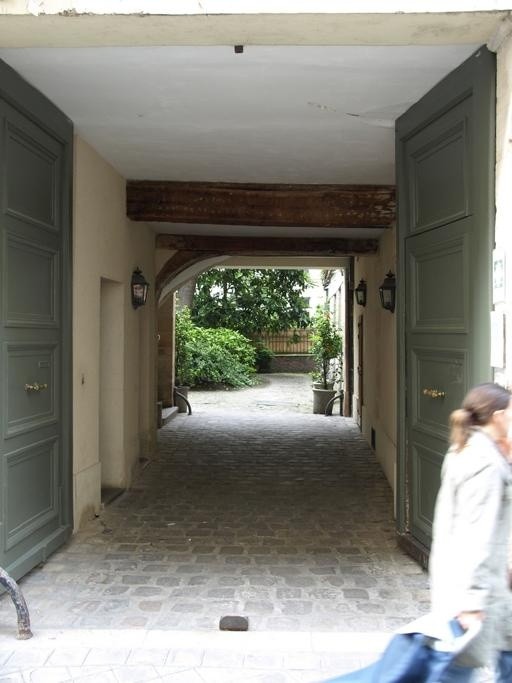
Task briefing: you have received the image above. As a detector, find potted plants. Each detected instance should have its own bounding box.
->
[175,304,195,413]
[307,307,341,414]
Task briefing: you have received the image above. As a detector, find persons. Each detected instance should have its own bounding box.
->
[429,383,512,683]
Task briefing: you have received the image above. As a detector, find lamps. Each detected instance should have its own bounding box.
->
[353,277,368,308]
[132,266,150,311]
[377,267,395,311]
